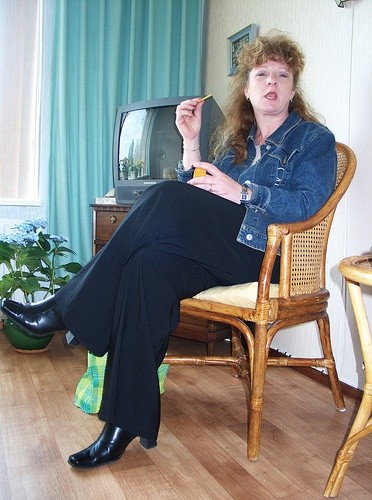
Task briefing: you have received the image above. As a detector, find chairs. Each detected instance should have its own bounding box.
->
[161,141,358,461]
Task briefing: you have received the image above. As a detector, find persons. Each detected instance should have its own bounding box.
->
[1,30,337,468]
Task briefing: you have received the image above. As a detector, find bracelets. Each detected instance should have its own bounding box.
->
[182,145,200,151]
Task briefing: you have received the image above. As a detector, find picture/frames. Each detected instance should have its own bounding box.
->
[226,23,258,77]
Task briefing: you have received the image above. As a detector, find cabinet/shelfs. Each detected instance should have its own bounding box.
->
[89,203,250,357]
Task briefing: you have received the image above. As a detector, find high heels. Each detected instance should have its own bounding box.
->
[0,294,79,345]
[68,422,158,468]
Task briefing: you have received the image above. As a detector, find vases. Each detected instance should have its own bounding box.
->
[4,317,54,354]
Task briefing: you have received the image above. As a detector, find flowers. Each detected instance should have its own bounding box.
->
[0,219,84,306]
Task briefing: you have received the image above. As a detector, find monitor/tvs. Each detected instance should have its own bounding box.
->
[113,96,226,205]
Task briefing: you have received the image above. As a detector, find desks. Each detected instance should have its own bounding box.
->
[323,253,372,498]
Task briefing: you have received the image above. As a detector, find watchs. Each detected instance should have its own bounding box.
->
[240,186,249,206]
